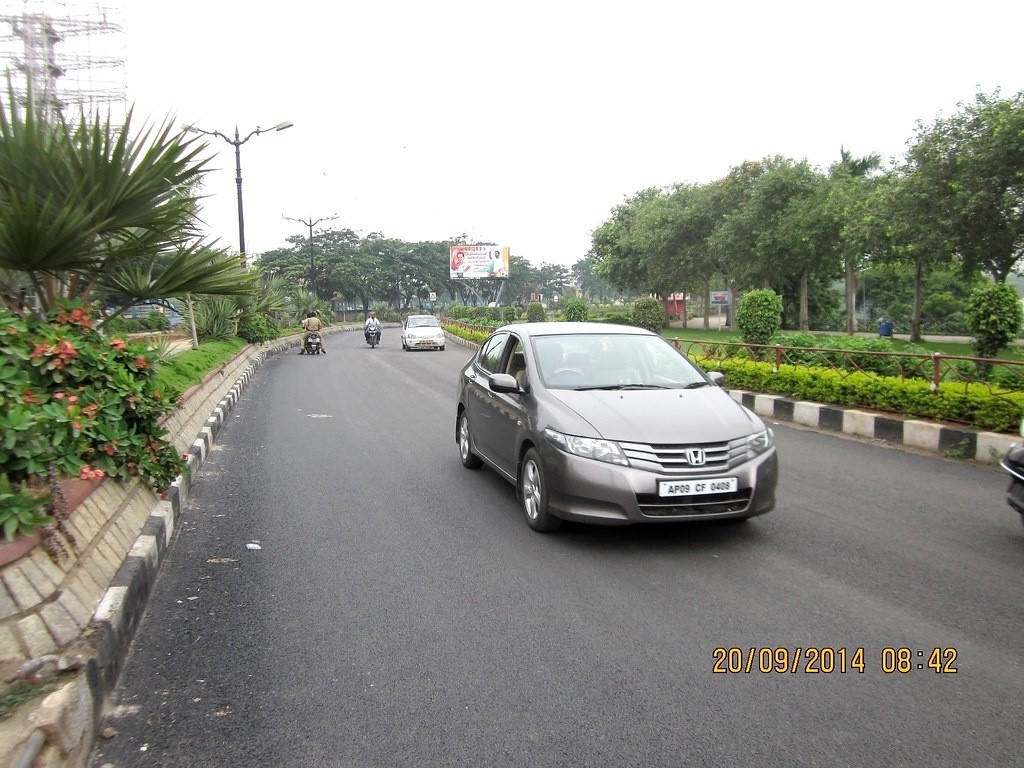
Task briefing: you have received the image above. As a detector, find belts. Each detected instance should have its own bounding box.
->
[308,330,319,331]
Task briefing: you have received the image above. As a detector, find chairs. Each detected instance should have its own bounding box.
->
[509,353,524,382]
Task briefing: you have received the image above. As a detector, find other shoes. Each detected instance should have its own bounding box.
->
[377,341,379,344]
[297,352,303,354]
[367,342,369,343]
[321,350,326,354]
[317,351,319,354]
[307,350,310,353]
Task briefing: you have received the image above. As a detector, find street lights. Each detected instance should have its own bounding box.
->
[181,119,294,272]
[282,215,341,284]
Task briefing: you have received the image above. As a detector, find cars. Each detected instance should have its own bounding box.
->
[455,322,780,536]
[401,315,445,351]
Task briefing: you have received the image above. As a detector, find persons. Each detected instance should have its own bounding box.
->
[298,312,326,354]
[363,312,381,342]
[489,248,503,273]
[453,252,471,272]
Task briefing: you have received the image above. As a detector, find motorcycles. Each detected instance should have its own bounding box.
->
[302,320,321,355]
[364,325,382,349]
